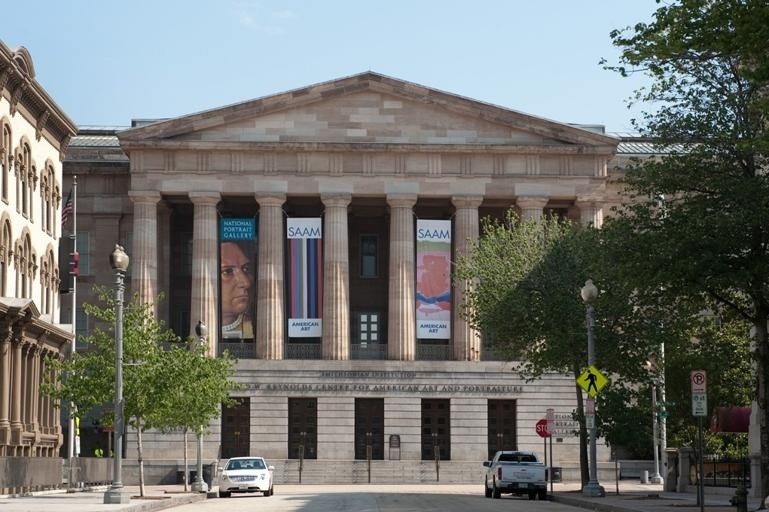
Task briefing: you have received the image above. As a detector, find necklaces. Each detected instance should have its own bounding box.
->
[222,313,244,331]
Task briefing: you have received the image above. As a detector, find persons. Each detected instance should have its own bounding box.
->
[220,240,255,339]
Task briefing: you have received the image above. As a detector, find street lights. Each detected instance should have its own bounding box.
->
[577,278,605,497]
[190,320,209,492]
[103,243,132,504]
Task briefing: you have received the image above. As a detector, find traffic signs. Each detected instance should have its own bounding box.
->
[552,428,580,436]
[552,421,580,429]
[552,412,579,422]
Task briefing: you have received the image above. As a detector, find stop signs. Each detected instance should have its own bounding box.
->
[536,419,552,437]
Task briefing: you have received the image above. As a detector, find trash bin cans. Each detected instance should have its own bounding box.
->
[176,470,208,485]
[545,467,562,483]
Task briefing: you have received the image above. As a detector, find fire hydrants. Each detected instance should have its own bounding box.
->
[729,482,749,512]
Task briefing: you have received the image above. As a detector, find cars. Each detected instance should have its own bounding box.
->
[217,456,275,497]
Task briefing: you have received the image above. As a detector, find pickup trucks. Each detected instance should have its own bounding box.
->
[482,450,547,500]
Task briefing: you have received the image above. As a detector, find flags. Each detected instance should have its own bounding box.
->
[62,190,74,229]
